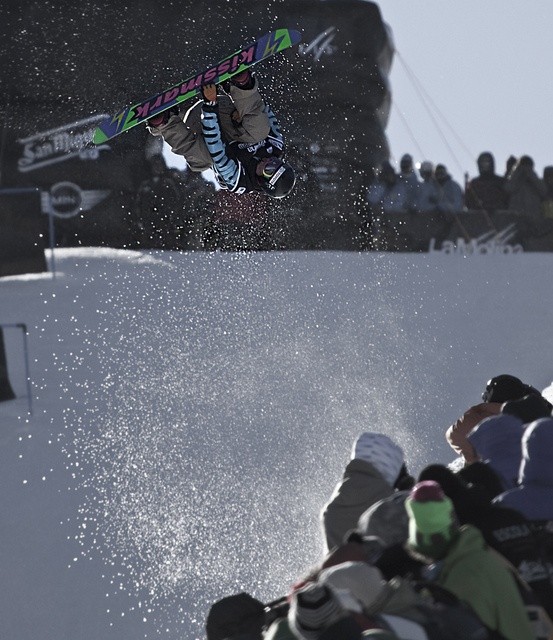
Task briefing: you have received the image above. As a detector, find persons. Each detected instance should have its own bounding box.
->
[145,69,296,199]
[505,156,548,216]
[206,375,553,640]
[416,162,434,214]
[432,165,464,213]
[541,167,552,215]
[465,152,508,210]
[394,153,417,212]
[505,156,516,179]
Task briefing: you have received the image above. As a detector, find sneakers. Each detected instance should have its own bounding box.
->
[148,105,180,133]
[225,70,250,87]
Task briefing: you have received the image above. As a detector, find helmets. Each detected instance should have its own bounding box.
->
[254,161,296,199]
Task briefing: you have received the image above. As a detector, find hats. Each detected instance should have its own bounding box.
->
[320,532,385,567]
[404,480,461,552]
[287,583,346,640]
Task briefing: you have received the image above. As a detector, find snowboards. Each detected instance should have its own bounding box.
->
[92,29,302,144]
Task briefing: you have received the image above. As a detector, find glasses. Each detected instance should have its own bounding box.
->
[262,158,282,177]
[485,379,501,392]
